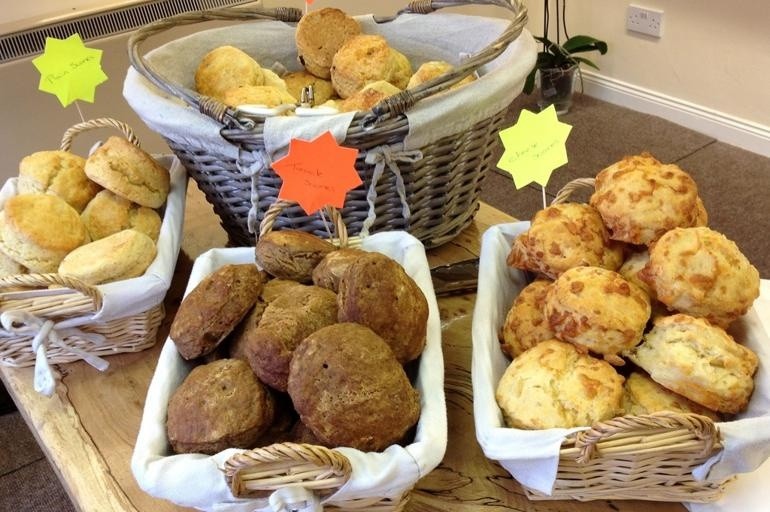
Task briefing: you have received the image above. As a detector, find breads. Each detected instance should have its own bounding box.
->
[624,370,721,425]
[85,135,171,208]
[498,274,552,360]
[495,337,625,431]
[290,418,324,446]
[58,229,156,286]
[310,247,369,292]
[167,358,275,454]
[544,266,652,367]
[243,284,339,392]
[589,152,709,244]
[195,7,475,116]
[0,192,91,273]
[334,252,430,365]
[629,313,759,415]
[648,225,761,330]
[80,189,162,242]
[16,149,102,214]
[288,323,420,452]
[169,264,263,360]
[620,247,659,304]
[506,202,627,279]
[254,229,340,282]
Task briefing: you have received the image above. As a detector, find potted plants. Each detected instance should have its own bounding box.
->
[522,35,607,115]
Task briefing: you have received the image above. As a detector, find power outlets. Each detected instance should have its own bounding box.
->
[626,4,664,38]
